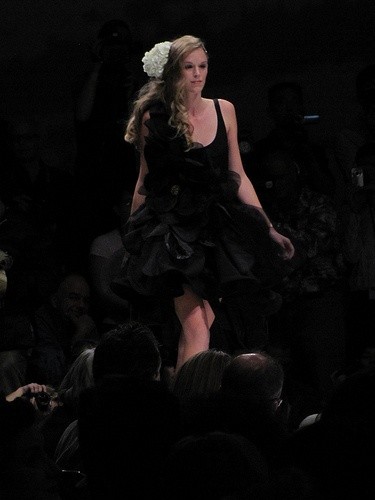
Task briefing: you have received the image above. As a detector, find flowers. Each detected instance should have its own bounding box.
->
[141,41,174,80]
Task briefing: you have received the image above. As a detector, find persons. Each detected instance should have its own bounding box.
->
[122,34,294,379]
[1,220,375,500]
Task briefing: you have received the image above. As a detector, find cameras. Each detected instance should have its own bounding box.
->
[36,393,51,412]
